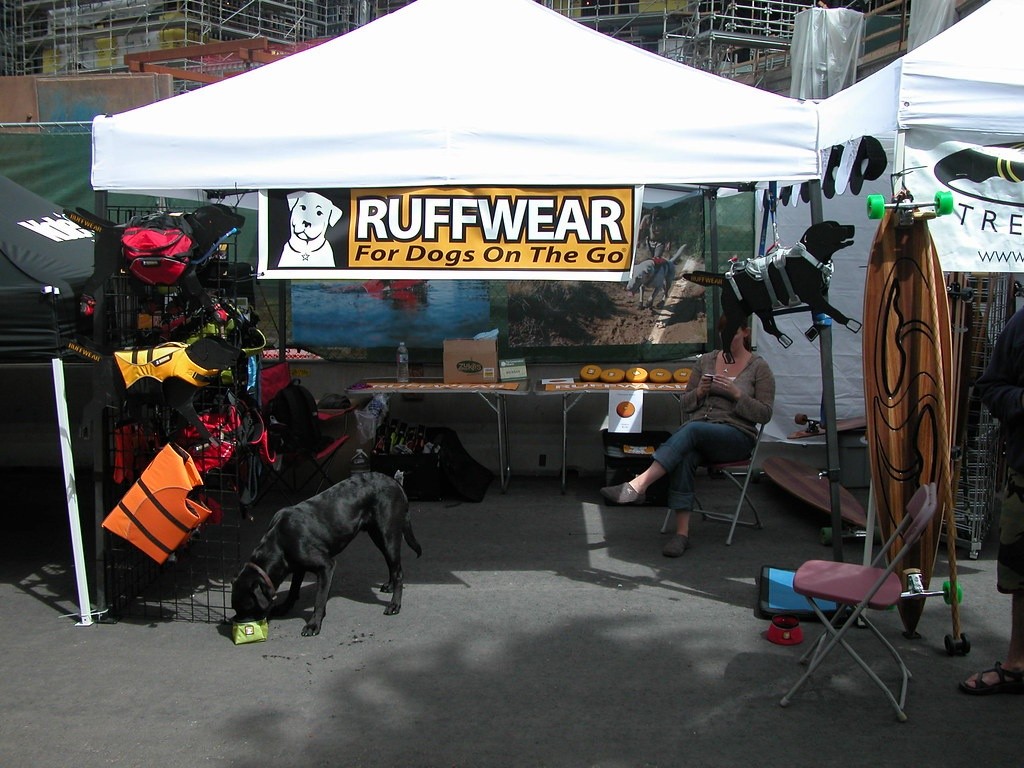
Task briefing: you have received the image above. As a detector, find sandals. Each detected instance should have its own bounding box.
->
[959,661,1024,696]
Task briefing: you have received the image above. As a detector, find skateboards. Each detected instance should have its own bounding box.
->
[863,187,965,636]
[754,453,882,549]
[784,411,864,440]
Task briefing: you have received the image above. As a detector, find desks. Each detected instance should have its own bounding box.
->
[345,380,532,493]
[532,379,686,494]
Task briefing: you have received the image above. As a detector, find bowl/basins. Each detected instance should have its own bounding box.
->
[767,615,802,645]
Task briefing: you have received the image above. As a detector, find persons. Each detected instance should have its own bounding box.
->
[637,206,671,296]
[601,314,774,556]
[960,308,1024,694]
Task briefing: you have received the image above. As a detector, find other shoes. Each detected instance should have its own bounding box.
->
[662,532,690,557]
[599,482,646,505]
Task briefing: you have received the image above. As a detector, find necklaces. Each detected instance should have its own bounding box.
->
[723,368,728,373]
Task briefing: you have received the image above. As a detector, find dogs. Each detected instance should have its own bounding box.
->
[231,471,423,636]
[63,203,245,313]
[683,221,863,365]
[66,337,240,436]
[626,243,687,310]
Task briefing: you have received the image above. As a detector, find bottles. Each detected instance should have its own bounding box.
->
[396,342,408,383]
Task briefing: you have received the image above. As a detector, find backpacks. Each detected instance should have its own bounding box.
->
[264,378,322,450]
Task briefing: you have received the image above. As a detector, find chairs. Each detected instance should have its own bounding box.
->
[259,360,356,507]
[780,482,938,722]
[660,421,765,546]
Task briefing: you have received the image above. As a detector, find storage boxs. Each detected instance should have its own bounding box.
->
[500,357,528,383]
[603,428,672,505]
[443,338,499,383]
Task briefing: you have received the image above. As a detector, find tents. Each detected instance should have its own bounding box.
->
[1,0,1024,625]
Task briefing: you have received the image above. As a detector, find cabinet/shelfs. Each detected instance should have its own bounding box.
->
[107,205,265,624]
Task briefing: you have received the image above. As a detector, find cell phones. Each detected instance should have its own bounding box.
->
[705,374,715,382]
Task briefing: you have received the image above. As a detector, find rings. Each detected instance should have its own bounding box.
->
[725,384,727,387]
